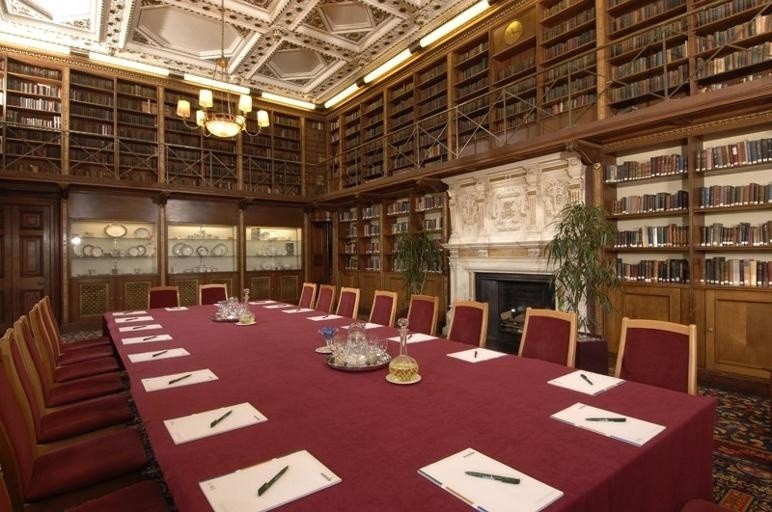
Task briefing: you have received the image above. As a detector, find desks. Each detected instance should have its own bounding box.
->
[104,299,714,510]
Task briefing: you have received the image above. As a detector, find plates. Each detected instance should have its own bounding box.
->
[236,322,256,326]
[69,222,302,277]
[386,374,424,385]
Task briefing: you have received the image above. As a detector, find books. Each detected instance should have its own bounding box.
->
[546,368,626,397]
[324,2,771,293]
[445,346,509,364]
[114,310,218,392]
[1,49,304,196]
[251,299,438,347]
[162,401,268,447]
[550,401,667,449]
[198,449,343,512]
[417,447,565,512]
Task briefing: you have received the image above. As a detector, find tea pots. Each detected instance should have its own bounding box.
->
[228,297,239,315]
[345,321,369,364]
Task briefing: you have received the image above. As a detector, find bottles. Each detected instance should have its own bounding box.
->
[389,319,418,382]
[238,288,256,323]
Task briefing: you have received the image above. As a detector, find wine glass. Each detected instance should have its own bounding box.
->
[321,327,337,351]
[328,336,388,365]
[216,300,245,317]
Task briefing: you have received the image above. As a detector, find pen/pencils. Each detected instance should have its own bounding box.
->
[586,417,626,422]
[133,326,146,329]
[169,374,192,384]
[210,410,232,427]
[144,336,156,341]
[465,472,520,484]
[153,351,167,357]
[475,351,477,358]
[126,317,138,321]
[257,466,288,496]
[581,374,592,385]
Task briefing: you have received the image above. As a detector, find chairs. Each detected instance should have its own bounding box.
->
[0,330,165,511]
